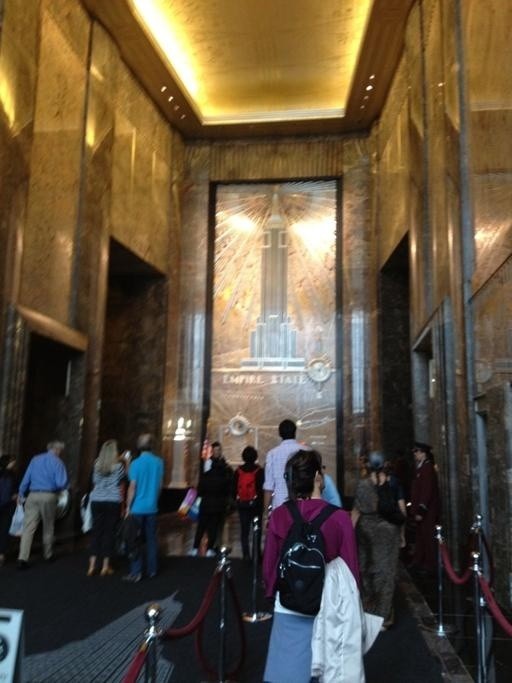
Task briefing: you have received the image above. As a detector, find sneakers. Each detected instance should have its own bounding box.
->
[17,562,26,568]
[101,568,113,576]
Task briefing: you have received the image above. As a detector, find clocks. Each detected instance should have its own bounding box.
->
[307,357,332,383]
[473,358,495,384]
[228,416,250,436]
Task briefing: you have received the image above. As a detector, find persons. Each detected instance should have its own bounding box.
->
[258,448,364,683]
[263,419,343,508]
[350,448,409,634]
[190,445,267,562]
[406,442,439,580]
[86,432,166,583]
[16,441,68,571]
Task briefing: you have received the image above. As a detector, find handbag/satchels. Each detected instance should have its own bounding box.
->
[79,493,93,534]
[377,481,406,527]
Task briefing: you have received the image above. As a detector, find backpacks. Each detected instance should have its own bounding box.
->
[277,502,339,616]
[237,465,260,502]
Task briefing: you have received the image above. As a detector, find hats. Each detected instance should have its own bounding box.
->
[412,441,432,453]
